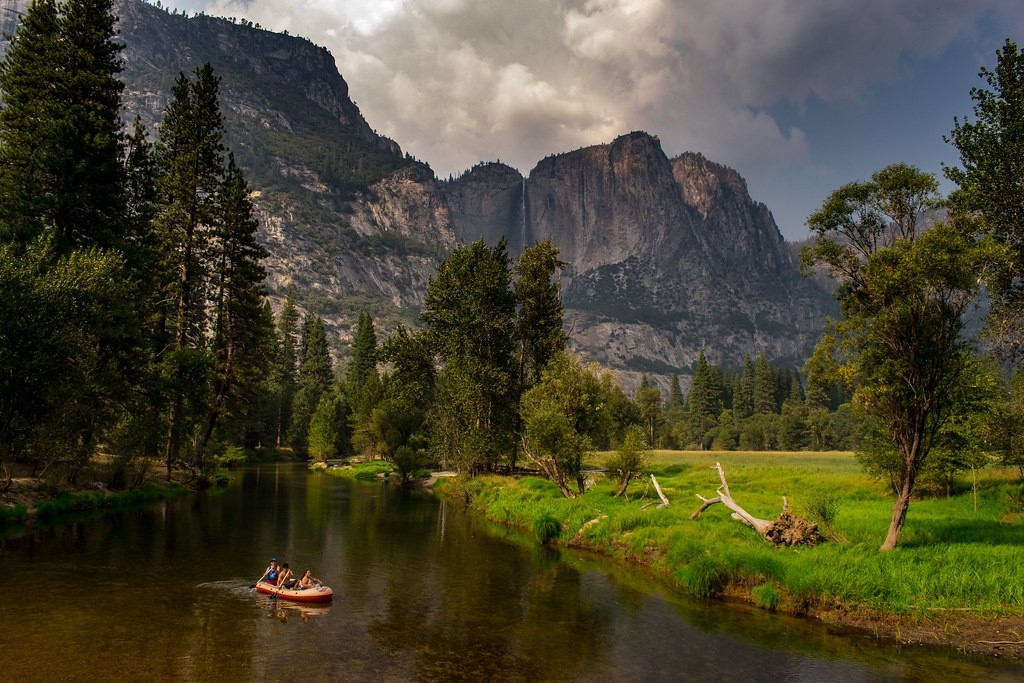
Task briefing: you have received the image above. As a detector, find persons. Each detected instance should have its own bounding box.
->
[263,558,280,585]
[301,569,322,590]
[277,563,301,589]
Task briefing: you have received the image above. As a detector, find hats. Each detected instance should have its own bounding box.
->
[270,558,276,562]
[283,563,288,568]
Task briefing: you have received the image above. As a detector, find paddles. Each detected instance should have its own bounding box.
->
[249,566,275,590]
[269,571,290,600]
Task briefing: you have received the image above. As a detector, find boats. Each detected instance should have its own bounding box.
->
[254,577,334,604]
